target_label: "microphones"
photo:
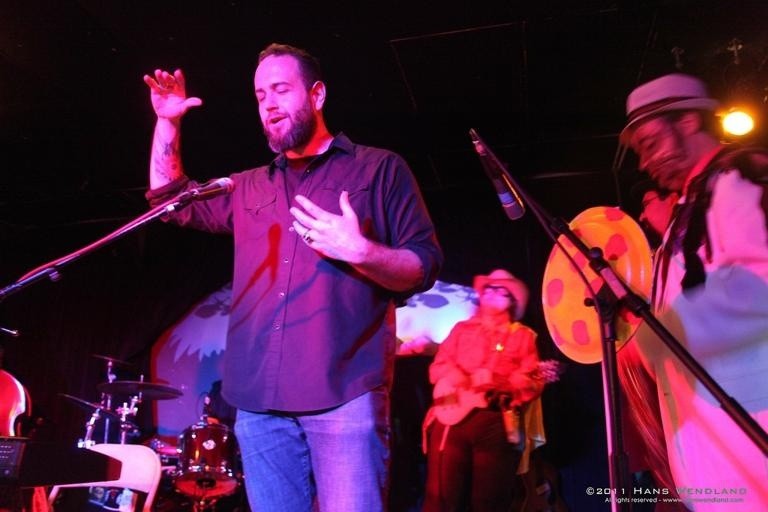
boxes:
[469,128,526,223]
[180,175,236,202]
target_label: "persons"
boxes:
[421,268,549,512]
[143,42,444,512]
[618,73,768,512]
[631,179,681,238]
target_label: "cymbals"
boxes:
[86,352,138,371]
[97,381,183,402]
[57,391,142,438]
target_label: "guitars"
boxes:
[432,360,560,427]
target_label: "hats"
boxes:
[473,269,530,320]
[620,74,722,144]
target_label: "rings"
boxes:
[302,233,316,246]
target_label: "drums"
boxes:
[149,439,188,500]
[174,422,244,498]
